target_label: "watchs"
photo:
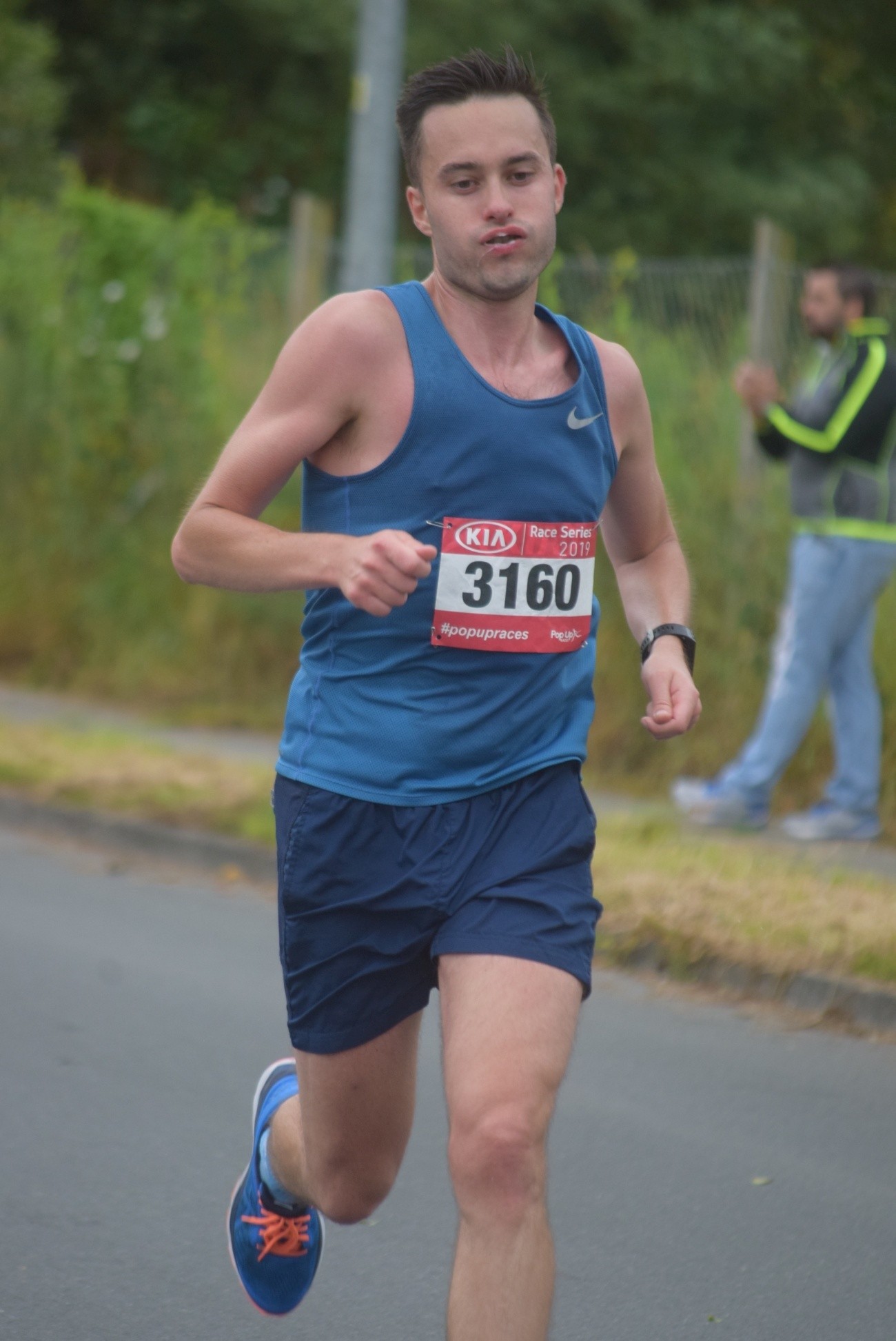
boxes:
[641,623,697,677]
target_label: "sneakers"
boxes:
[224,1058,325,1315]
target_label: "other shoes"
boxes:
[781,803,881,841]
[670,780,766,831]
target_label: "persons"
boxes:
[171,55,702,1341]
[675,264,896,841]
